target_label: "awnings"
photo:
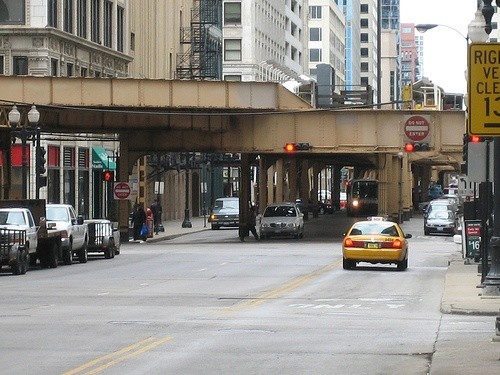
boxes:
[92,147,116,171]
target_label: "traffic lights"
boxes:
[101,171,114,182]
[405,143,431,152]
[35,146,46,174]
[469,136,483,143]
[285,143,310,151]
[38,175,47,187]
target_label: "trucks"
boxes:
[0,200,68,276]
[345,178,379,218]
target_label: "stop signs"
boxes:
[404,116,429,142]
[113,182,131,200]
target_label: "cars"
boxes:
[259,203,305,240]
[342,216,412,271]
[81,219,122,256]
[420,186,461,236]
[208,196,240,230]
[293,190,347,218]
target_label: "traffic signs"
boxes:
[457,177,480,197]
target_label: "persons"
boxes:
[240,208,260,241]
[150,199,162,235]
[130,204,147,241]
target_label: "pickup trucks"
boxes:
[46,205,91,266]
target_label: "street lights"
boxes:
[468,1,496,273]
[0,102,41,200]
